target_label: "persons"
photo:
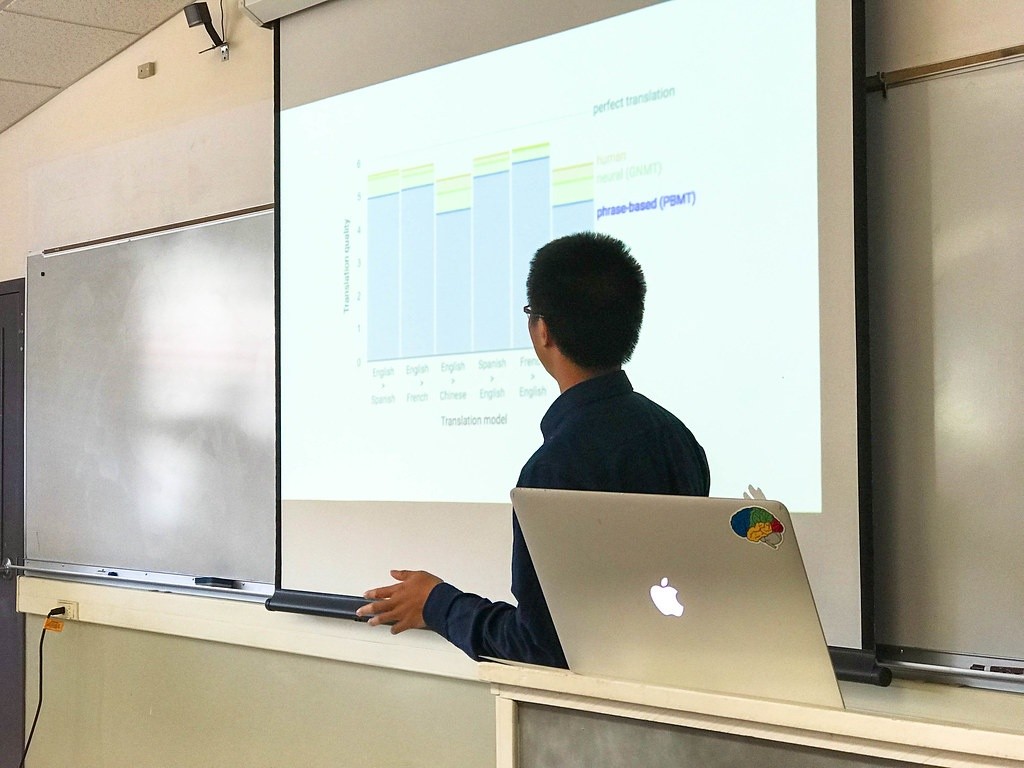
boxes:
[356,234,711,669]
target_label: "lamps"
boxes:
[182,1,229,55]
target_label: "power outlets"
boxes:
[137,61,154,79]
[56,599,79,622]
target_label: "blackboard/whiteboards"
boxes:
[22,202,280,596]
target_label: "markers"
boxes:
[190,575,246,589]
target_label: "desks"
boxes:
[485,662,1024,768]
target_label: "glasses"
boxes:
[524,303,547,322]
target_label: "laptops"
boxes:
[509,487,846,712]
